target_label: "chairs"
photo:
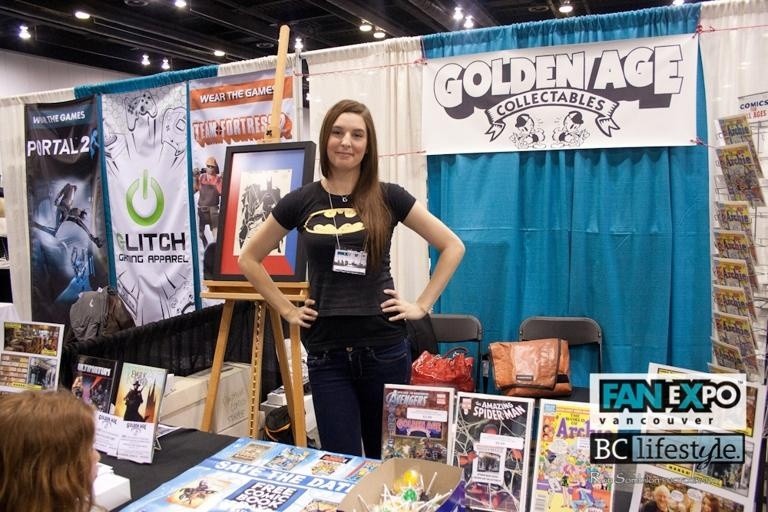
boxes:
[519,317,604,475]
[431,315,483,392]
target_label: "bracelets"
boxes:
[416,301,431,316]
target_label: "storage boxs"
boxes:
[186,360,252,433]
[158,376,208,429]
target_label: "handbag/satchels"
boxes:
[487,337,572,400]
[409,346,475,394]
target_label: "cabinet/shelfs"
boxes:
[711,120,768,385]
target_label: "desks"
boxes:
[98,429,239,512]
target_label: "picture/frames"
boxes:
[213,141,316,281]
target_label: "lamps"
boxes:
[174,0,186,8]
[142,53,150,67]
[161,56,170,71]
[19,24,31,40]
[295,36,303,49]
[360,6,474,40]
[558,0,573,13]
[213,50,227,57]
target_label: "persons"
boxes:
[238,99,466,459]
[0,389,99,511]
[191,157,223,247]
[123,380,146,422]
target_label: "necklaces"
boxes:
[324,176,358,203]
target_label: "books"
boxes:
[713,110,766,360]
[113,362,169,453]
[69,352,119,413]
[380,363,766,510]
